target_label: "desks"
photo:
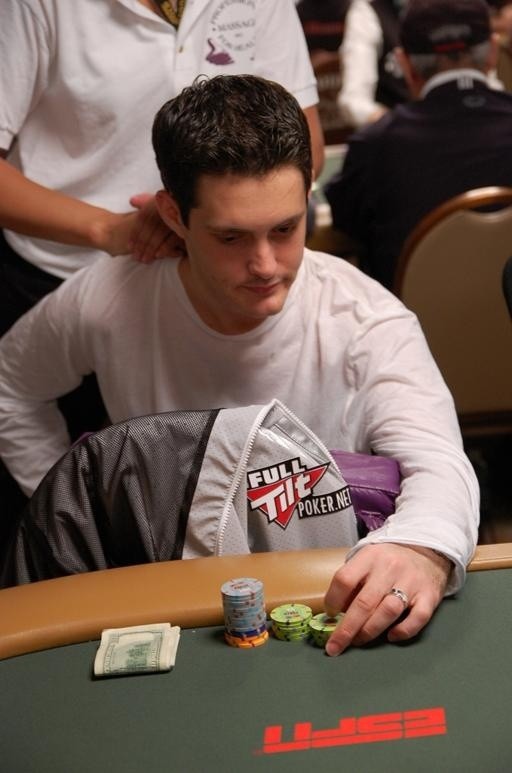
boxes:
[305,141,369,258]
[1,542,512,772]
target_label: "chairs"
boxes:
[391,184,511,439]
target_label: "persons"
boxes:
[313,0,512,326]
[0,68,484,661]
[0,0,327,583]
[332,0,500,131]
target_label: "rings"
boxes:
[391,586,412,610]
